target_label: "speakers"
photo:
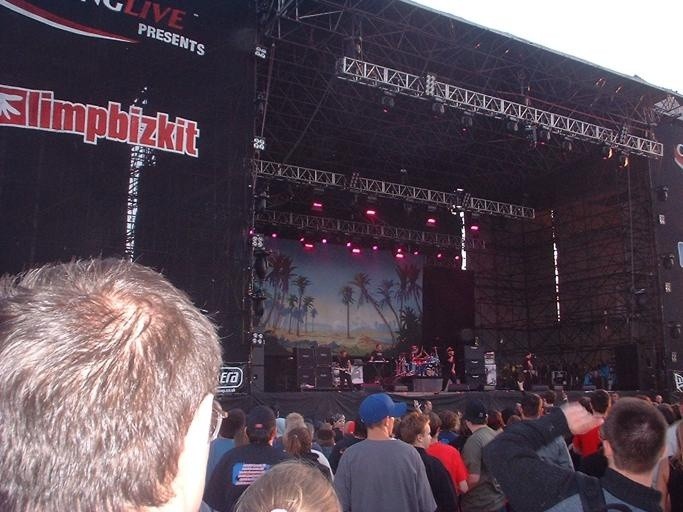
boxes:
[616,342,649,391]
[240,344,264,393]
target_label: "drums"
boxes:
[408,362,419,375]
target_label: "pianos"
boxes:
[368,360,389,365]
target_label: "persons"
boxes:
[421,411,468,495]
[652,402,683,488]
[431,344,440,377]
[634,394,682,425]
[409,343,417,375]
[440,345,457,392]
[497,350,579,391]
[334,392,437,512]
[207,400,228,442]
[1,256,223,512]
[487,406,506,435]
[482,397,668,512]
[332,417,366,476]
[305,413,331,431]
[274,411,308,451]
[439,412,467,451]
[413,399,423,414]
[656,418,682,512]
[368,343,386,391]
[328,414,346,443]
[203,404,294,512]
[328,420,355,475]
[572,389,612,478]
[388,418,402,438]
[553,383,568,405]
[205,408,246,489]
[579,361,619,390]
[305,431,323,454]
[517,380,526,396]
[457,407,466,419]
[424,399,433,413]
[518,392,574,469]
[507,415,520,426]
[400,412,461,512]
[283,426,334,484]
[514,399,523,414]
[501,406,521,426]
[610,391,621,403]
[235,460,342,512]
[317,421,335,460]
[461,398,507,511]
[331,350,356,394]
[270,404,280,418]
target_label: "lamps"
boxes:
[250,331,264,346]
[253,43,268,62]
[252,135,265,150]
[540,131,550,144]
[460,115,472,131]
[618,155,628,168]
[250,233,264,248]
[600,146,613,160]
[380,95,394,112]
[432,102,444,118]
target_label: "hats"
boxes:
[465,399,485,418]
[247,406,275,430]
[359,393,407,423]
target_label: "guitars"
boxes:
[333,367,352,375]
